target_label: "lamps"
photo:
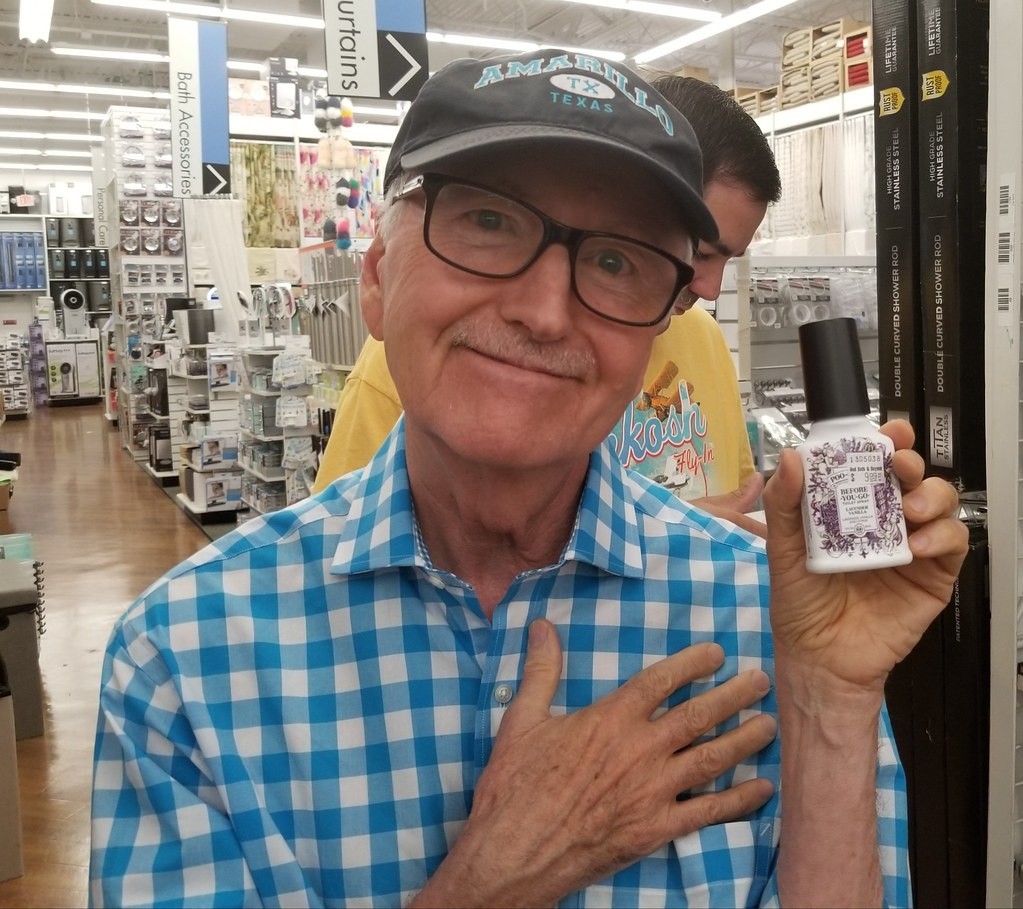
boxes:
[0,0,800,172]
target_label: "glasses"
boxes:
[390,175,695,328]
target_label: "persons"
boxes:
[212,483,225,500]
[88,51,970,909]
[208,442,221,458]
[215,364,229,379]
[311,76,780,543]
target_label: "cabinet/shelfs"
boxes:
[117,320,150,461]
[0,214,114,316]
[169,338,235,515]
[136,338,181,479]
[236,333,331,517]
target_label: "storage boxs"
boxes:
[669,18,873,121]
[0,332,35,410]
[0,482,12,511]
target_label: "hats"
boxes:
[381,48,721,245]
